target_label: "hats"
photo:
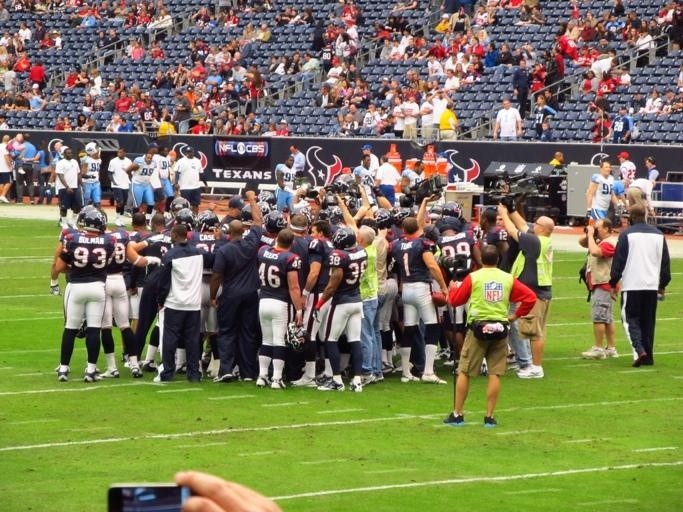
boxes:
[361,145,372,150]
[229,197,245,208]
[616,150,629,159]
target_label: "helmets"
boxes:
[331,225,356,249]
[290,201,343,225]
[242,191,288,233]
[284,322,310,353]
[171,196,219,231]
[84,143,100,155]
[78,205,108,233]
[373,207,412,229]
[431,201,463,219]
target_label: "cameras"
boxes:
[324,185,340,193]
[584,227,597,237]
[438,255,467,274]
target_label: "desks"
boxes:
[444,190,484,224]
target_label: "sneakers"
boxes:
[122,353,164,382]
[581,346,653,367]
[57,215,77,231]
[421,374,447,384]
[256,374,287,388]
[400,372,420,382]
[444,412,465,426]
[202,352,242,383]
[484,418,496,428]
[290,372,345,390]
[381,360,414,373]
[516,364,544,378]
[433,347,460,375]
[56,364,71,380]
[83,367,100,382]
[114,212,151,230]
[349,375,384,392]
[98,368,119,378]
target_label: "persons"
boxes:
[50,176,553,393]
[1,1,683,233]
[172,468,284,512]
[441,244,540,427]
[578,205,672,368]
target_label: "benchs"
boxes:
[200,179,247,205]
[257,181,278,199]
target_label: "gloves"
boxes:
[49,278,60,295]
[312,309,321,323]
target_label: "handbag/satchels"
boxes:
[472,321,509,340]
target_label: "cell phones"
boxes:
[108,483,191,512]
